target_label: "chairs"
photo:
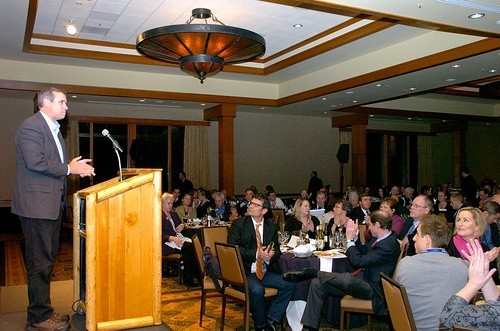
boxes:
[162,208,417,331]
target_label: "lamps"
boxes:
[135,8,266,85]
[66,19,77,35]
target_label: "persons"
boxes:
[325,198,358,242]
[173,172,193,203]
[307,171,324,195]
[161,180,500,331]
[11,87,95,331]
[363,198,405,240]
[284,198,320,240]
[460,166,478,202]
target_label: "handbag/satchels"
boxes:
[282,267,317,281]
[202,247,222,280]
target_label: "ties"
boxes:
[404,224,415,238]
[351,240,378,276]
[255,224,264,280]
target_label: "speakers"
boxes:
[336,143,350,163]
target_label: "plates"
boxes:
[312,251,335,258]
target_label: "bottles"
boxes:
[314,223,329,250]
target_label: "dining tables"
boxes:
[180,220,233,279]
[268,242,348,331]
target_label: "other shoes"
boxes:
[185,276,195,287]
[255,320,276,331]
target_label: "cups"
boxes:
[278,230,347,253]
[182,216,225,227]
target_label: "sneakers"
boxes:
[51,312,70,322]
[26,318,71,331]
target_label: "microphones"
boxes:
[101,128,124,153]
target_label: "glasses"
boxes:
[410,202,427,209]
[248,201,266,209]
